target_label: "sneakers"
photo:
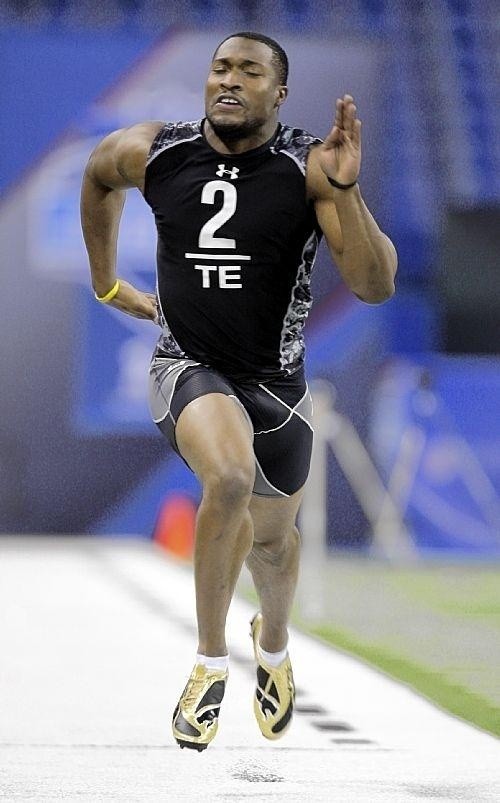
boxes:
[249,609,296,743]
[170,662,230,753]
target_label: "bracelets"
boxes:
[89,278,121,303]
[328,176,358,189]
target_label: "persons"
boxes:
[76,31,402,754]
